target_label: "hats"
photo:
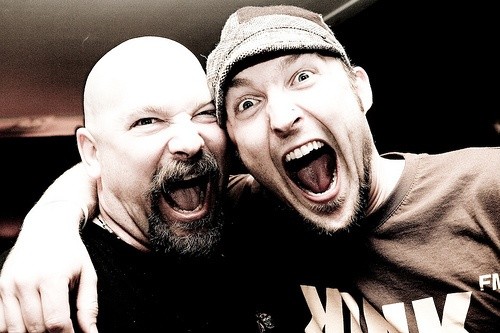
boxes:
[204,5,353,129]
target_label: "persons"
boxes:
[1,5,499,333]
[69,36,295,333]
